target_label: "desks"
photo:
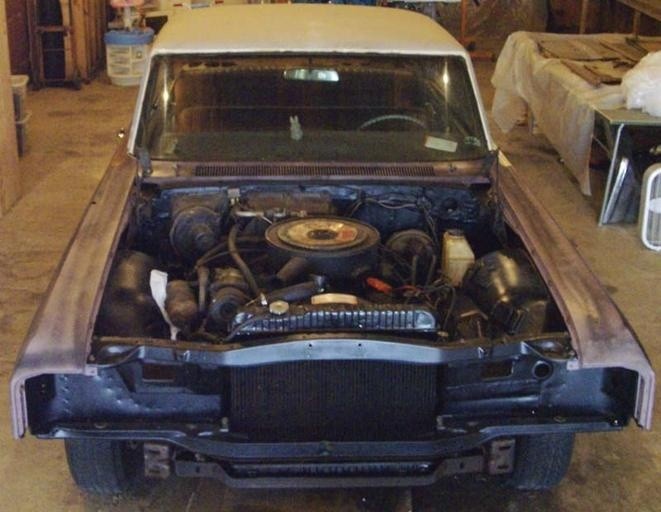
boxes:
[517,32,660,226]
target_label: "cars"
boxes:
[11,2,657,496]
